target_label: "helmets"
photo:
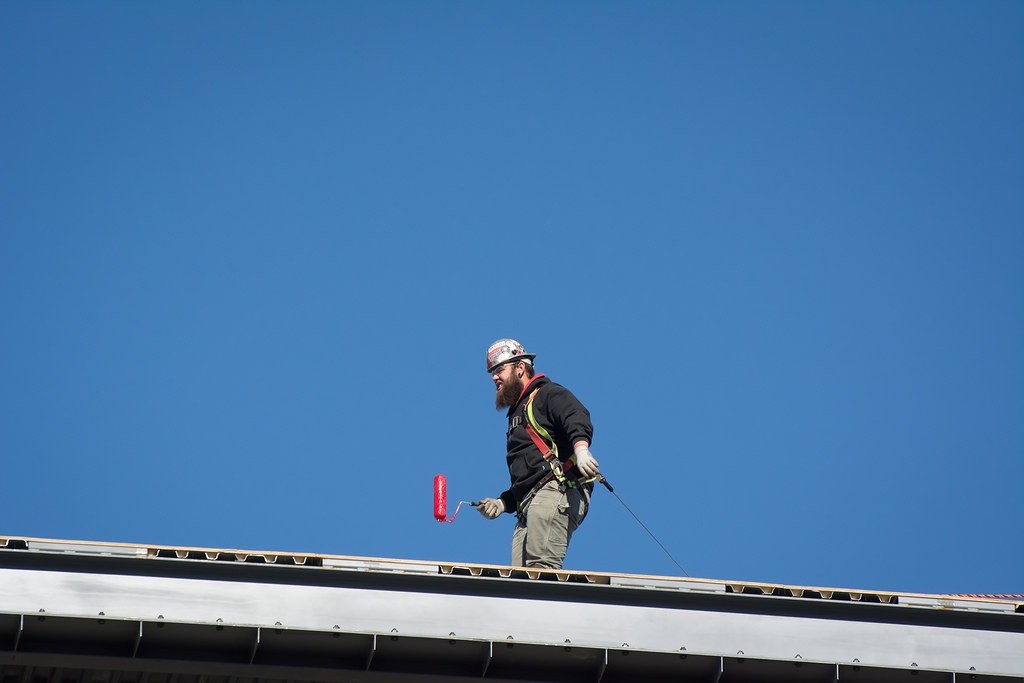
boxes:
[486,339,535,372]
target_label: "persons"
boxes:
[477,338,599,570]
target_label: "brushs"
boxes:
[432,473,481,523]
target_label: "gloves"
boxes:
[574,445,599,479]
[476,498,507,520]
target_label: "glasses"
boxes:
[492,362,516,375]
